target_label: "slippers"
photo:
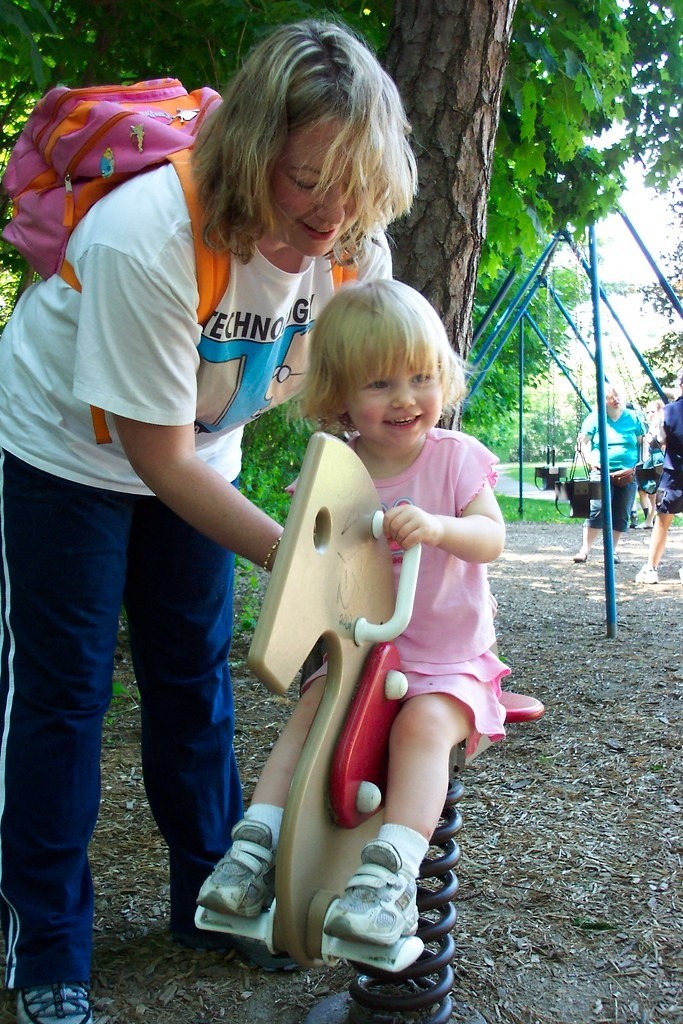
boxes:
[635,523,655,528]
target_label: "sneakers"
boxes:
[636,564,659,584]
[196,819,278,918]
[16,981,94,1024]
[324,839,419,947]
[174,932,300,970]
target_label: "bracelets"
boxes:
[261,532,290,575]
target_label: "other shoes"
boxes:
[613,553,620,563]
[573,552,588,562]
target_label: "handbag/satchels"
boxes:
[593,466,638,488]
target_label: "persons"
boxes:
[0,14,421,1024]
[198,277,507,946]
[634,377,683,587]
[571,383,647,569]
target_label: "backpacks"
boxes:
[0,77,359,445]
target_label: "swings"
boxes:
[534,261,566,492]
[554,225,602,521]
[574,265,665,494]
